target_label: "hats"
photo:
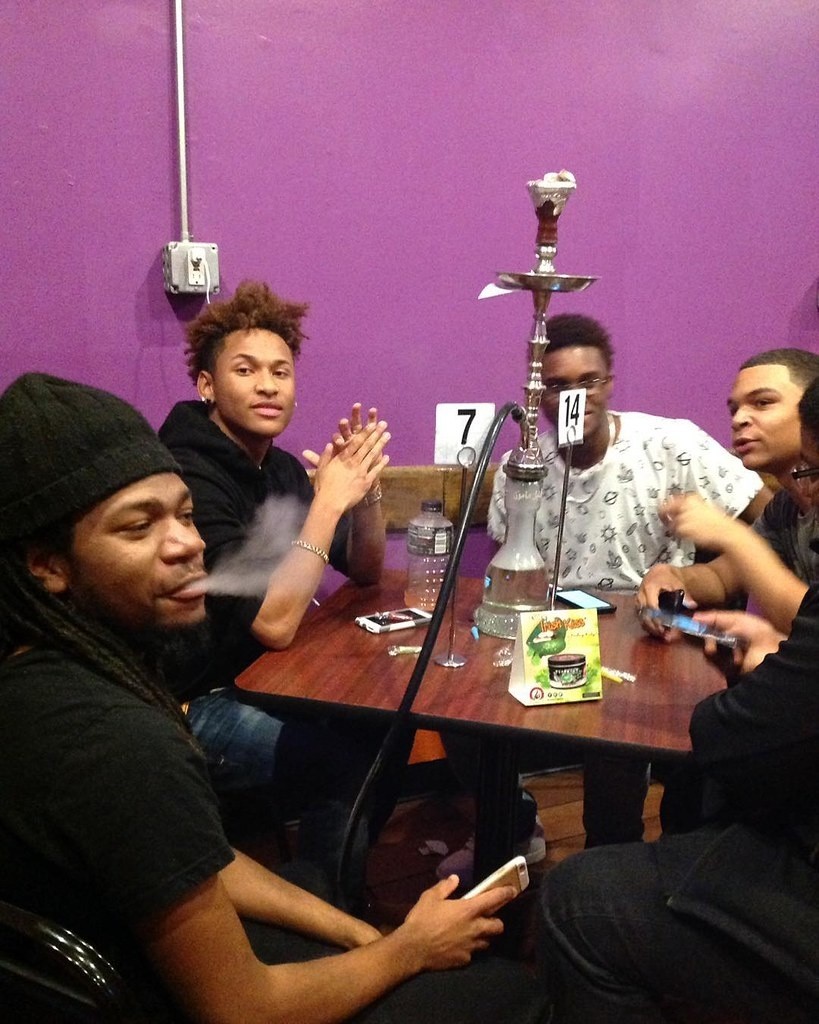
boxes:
[1,371,183,567]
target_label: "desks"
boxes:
[233,573,729,899]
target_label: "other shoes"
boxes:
[331,873,377,919]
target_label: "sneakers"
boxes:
[436,814,547,881]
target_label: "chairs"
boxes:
[0,902,143,1024]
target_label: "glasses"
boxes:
[540,376,613,403]
[790,461,819,489]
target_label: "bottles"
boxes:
[404,500,453,613]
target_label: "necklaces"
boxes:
[567,412,616,503]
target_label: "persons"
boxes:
[435,309,771,887]
[158,278,419,913]
[0,370,520,1024]
[535,374,819,1024]
[634,348,819,664]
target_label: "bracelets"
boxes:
[353,485,382,509]
[291,540,329,564]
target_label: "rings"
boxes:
[664,513,672,522]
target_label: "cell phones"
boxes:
[353,607,434,634]
[457,855,530,916]
[658,589,685,630]
[662,612,737,649]
[550,588,617,615]
